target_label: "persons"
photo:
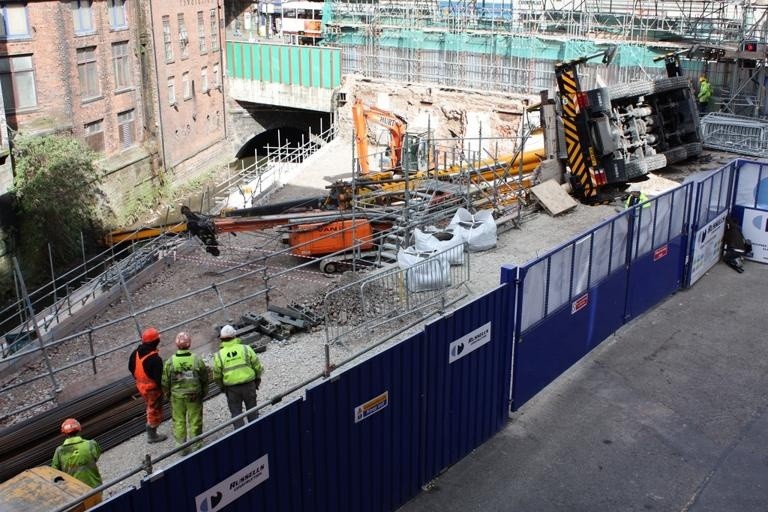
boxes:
[159,332,208,456]
[51,418,103,497]
[212,324,263,430]
[128,327,168,444]
[698,76,714,117]
[751,66,768,118]
[624,183,651,211]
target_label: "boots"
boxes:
[146,424,204,456]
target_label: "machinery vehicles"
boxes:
[290,98,435,273]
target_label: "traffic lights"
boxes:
[741,41,757,69]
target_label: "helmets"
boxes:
[628,183,642,192]
[61,418,81,433]
[175,331,192,348]
[221,325,236,338]
[142,328,160,344]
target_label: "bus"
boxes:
[282,3,431,45]
